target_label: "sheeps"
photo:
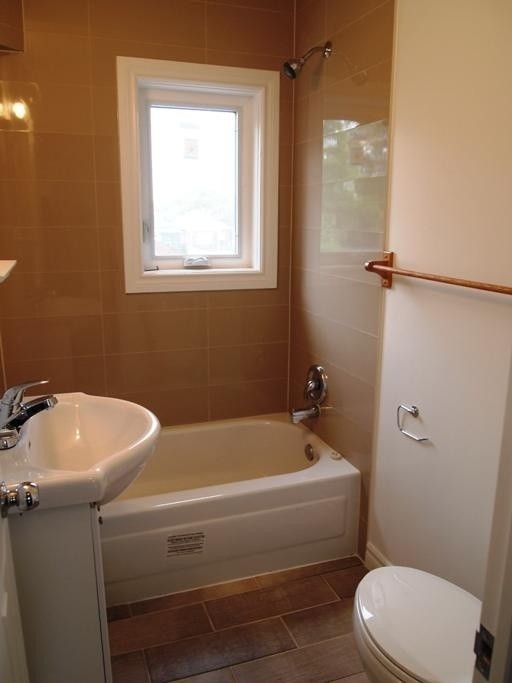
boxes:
[289,403,320,425]
[1,380,58,447]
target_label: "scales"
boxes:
[100,411,361,622]
